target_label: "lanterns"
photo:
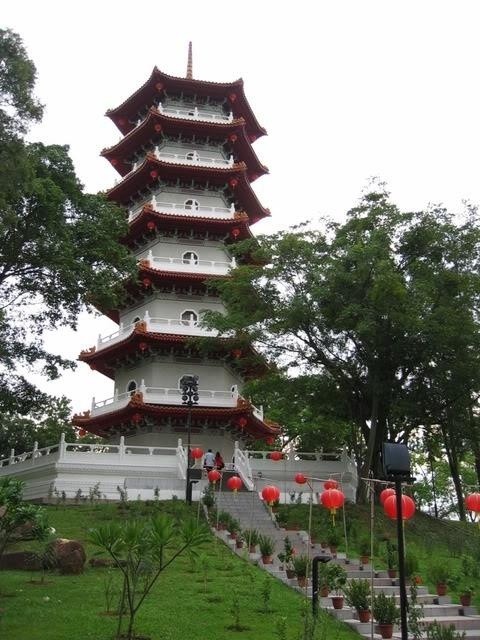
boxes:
[295,472,306,484]
[106,112,128,170]
[324,480,338,490]
[208,470,221,484]
[262,485,280,506]
[227,474,242,494]
[265,432,277,447]
[384,495,416,520]
[321,489,344,527]
[271,451,281,460]
[131,80,168,426]
[250,128,265,228]
[75,426,90,438]
[226,83,249,445]
[466,491,479,512]
[380,488,396,504]
[192,448,202,459]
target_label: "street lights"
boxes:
[379,442,411,640]
[179,374,199,504]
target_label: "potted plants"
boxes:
[316,560,336,597]
[258,535,278,564]
[271,498,301,533]
[275,537,296,578]
[329,564,348,610]
[459,585,474,607]
[409,561,421,587]
[310,520,341,554]
[359,542,372,565]
[205,486,239,539]
[372,590,467,637]
[235,529,246,549]
[243,527,260,553]
[291,554,312,588]
[387,546,399,578]
[344,578,373,623]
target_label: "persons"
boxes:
[214,452,224,470]
[204,449,215,471]
